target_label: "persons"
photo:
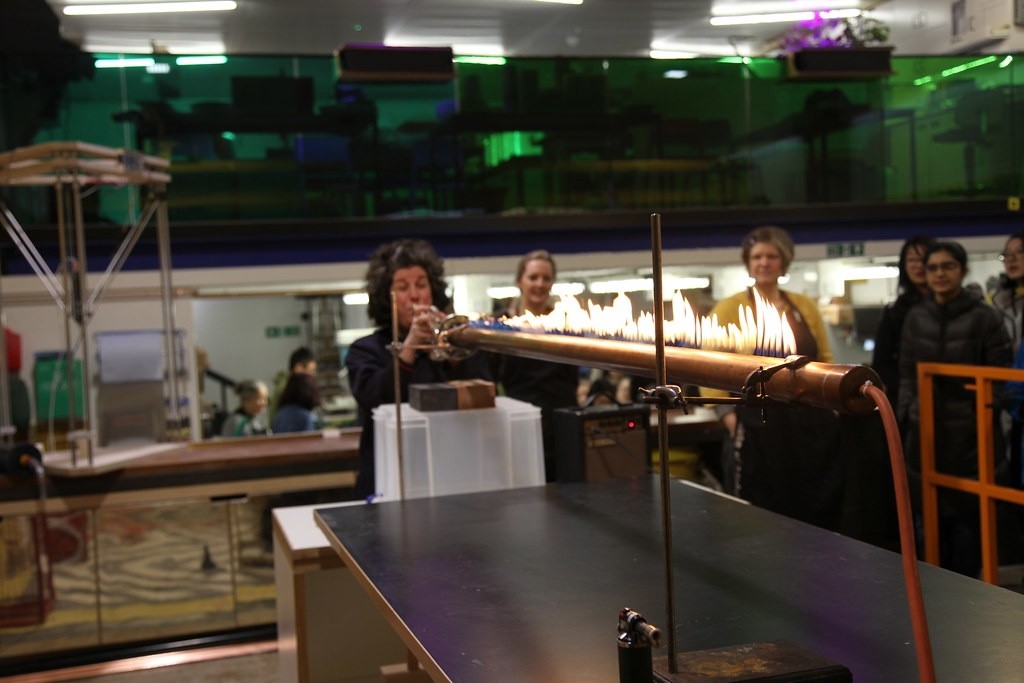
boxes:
[474,248,580,488]
[271,372,323,434]
[896,240,1014,575]
[982,230,1024,566]
[220,377,274,437]
[584,367,630,410]
[345,239,499,501]
[289,346,317,376]
[697,226,836,498]
[867,232,940,546]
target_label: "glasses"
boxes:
[927,263,959,271]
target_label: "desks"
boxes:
[272,500,413,683]
[316,477,1024,683]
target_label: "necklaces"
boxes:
[516,298,551,319]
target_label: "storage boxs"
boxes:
[373,396,545,502]
